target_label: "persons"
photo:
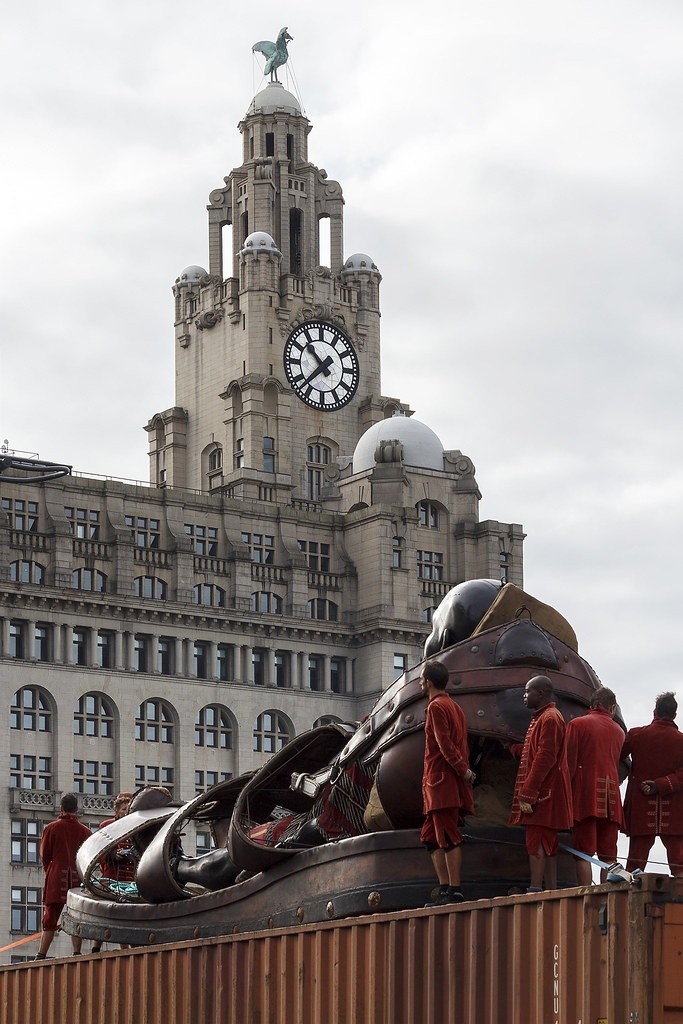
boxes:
[93,792,140,953]
[33,793,93,962]
[127,579,630,892]
[419,661,478,908]
[509,676,575,895]
[622,690,683,876]
[568,687,625,887]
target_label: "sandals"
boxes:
[57,605,631,947]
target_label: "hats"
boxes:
[115,792,133,803]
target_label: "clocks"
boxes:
[284,319,360,412]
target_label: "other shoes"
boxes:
[442,890,463,904]
[92,947,100,953]
[72,952,82,956]
[34,952,46,961]
[425,892,446,906]
